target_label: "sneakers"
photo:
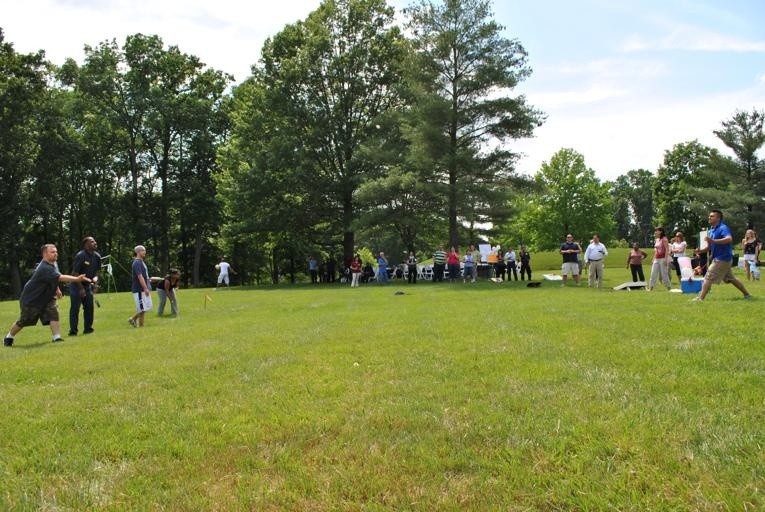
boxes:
[127,317,137,327]
[689,296,704,301]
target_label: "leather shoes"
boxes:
[4,337,14,347]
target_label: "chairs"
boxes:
[363,260,466,281]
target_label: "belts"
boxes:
[588,258,602,262]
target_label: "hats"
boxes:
[134,244,146,254]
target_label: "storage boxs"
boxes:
[681,278,705,293]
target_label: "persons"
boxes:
[626,242,648,282]
[649,209,762,301]
[305,244,531,287]
[156,268,181,316]
[215,260,238,287]
[128,245,153,327]
[68,236,101,336]
[559,233,609,289]
[4,244,92,346]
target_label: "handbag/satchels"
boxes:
[752,270,760,280]
